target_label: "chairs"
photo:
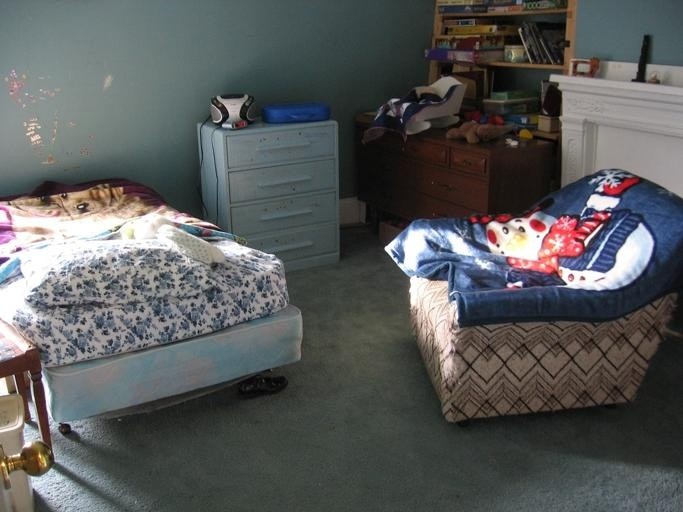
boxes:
[407,166,682,425]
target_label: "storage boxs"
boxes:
[536,114,559,132]
[503,44,530,63]
[379,212,413,249]
[481,97,539,116]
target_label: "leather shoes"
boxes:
[237,375,288,399]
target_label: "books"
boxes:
[424,0,567,65]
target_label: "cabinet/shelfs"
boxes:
[195,119,340,276]
[424,1,578,118]
[353,110,556,226]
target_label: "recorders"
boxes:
[210,94,257,128]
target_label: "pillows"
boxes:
[18,239,202,309]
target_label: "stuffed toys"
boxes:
[446,122,517,144]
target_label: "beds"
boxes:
[2,179,305,435]
